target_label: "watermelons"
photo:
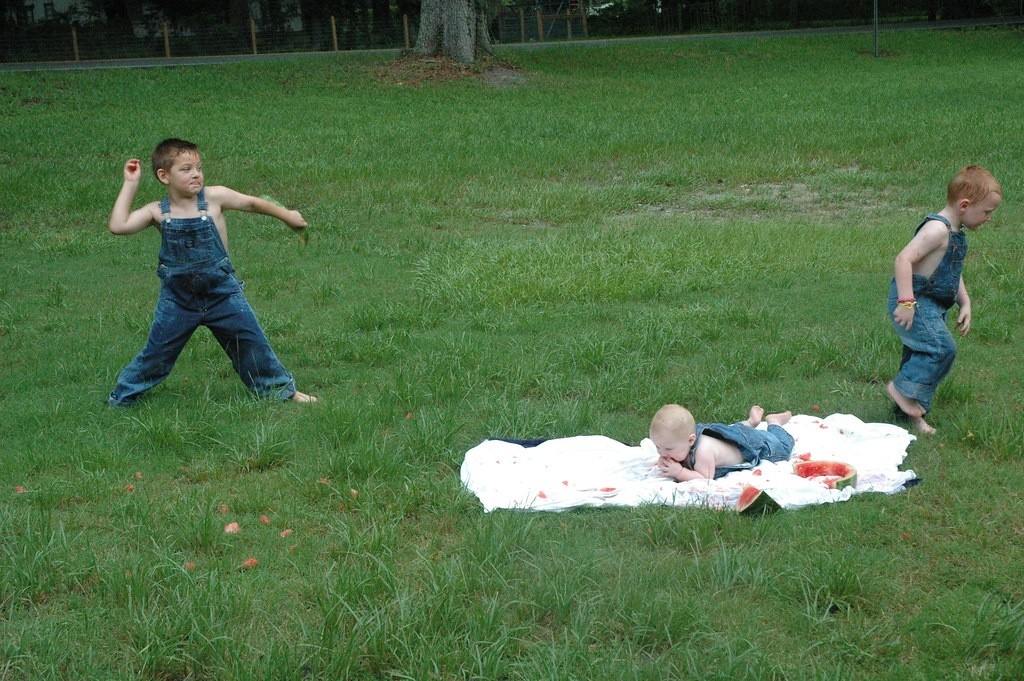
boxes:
[15,472,359,571]
[738,484,782,514]
[538,481,616,498]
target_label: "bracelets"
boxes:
[897,297,918,309]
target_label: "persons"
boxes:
[886,164,1002,436]
[108,138,317,405]
[650,403,794,482]
